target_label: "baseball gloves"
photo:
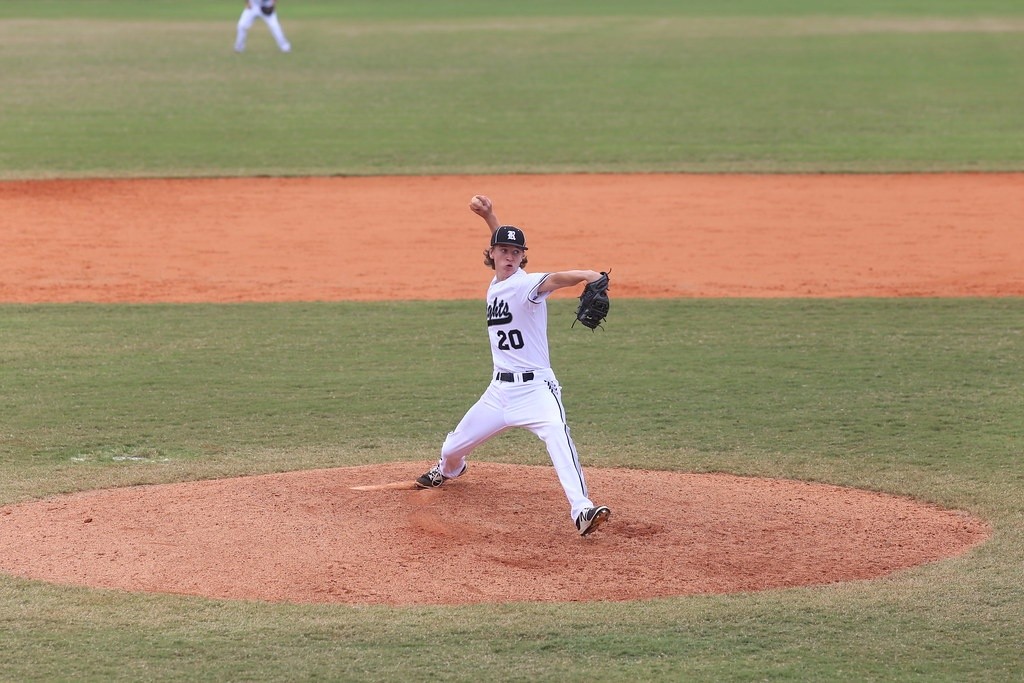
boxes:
[571,268,611,332]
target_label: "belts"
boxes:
[496,372,534,382]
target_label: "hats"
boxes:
[490,225,528,250]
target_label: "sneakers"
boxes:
[415,462,467,488]
[575,505,610,536]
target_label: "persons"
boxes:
[416,195,610,538]
[234,0,291,54]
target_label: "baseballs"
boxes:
[471,196,484,210]
